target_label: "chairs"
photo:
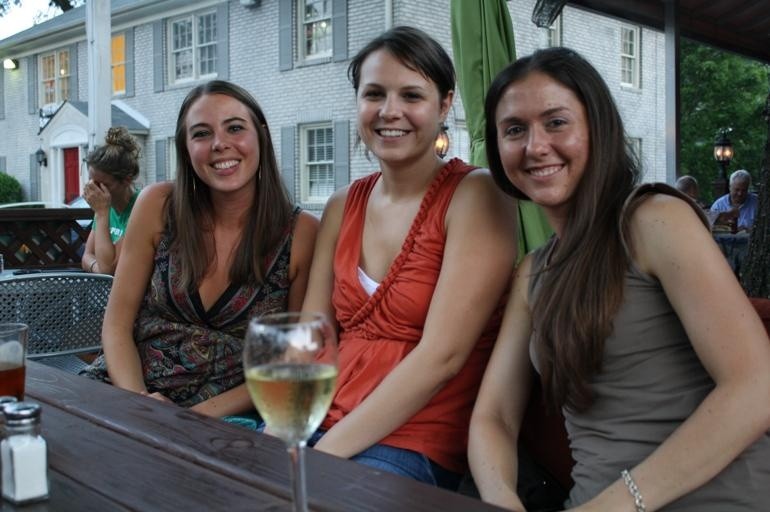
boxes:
[748,297,770,337]
[0,274,113,378]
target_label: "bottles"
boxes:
[0,395,51,507]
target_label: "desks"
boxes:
[710,228,752,271]
[0,358,534,512]
[0,268,93,330]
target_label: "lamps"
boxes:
[3,58,18,71]
[35,146,47,167]
[83,142,97,170]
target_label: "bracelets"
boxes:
[91,260,96,273]
[619,469,646,512]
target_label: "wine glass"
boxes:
[243,311,338,512]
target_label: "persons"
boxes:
[74,81,323,434]
[74,125,145,363]
[255,24,521,487]
[708,169,759,272]
[465,44,769,511]
[675,175,740,225]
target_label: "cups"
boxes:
[0,322,29,402]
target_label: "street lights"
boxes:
[713,129,735,200]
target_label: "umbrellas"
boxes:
[449,0,566,268]
